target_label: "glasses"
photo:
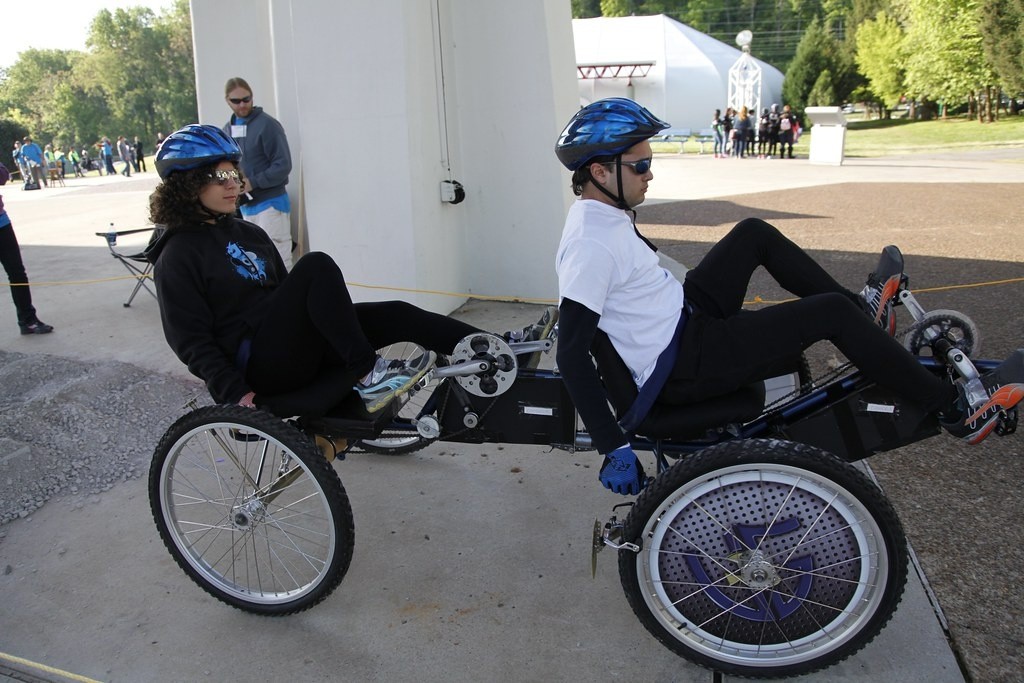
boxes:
[586,157,652,174]
[209,167,240,185]
[229,96,251,104]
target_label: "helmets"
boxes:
[556,96,672,171]
[154,123,243,179]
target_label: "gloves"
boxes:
[599,442,647,494]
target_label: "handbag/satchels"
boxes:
[24,180,40,190]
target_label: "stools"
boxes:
[46,167,66,188]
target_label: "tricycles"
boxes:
[146,273,1023,679]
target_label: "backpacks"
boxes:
[780,117,791,130]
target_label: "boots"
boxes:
[780,149,784,158]
[788,147,795,158]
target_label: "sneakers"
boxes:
[505,306,559,368]
[859,244,904,338]
[939,350,1024,444]
[21,321,53,335]
[353,350,437,413]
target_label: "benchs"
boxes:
[647,137,688,153]
[695,138,715,154]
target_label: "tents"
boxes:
[572,14,785,137]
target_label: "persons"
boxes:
[223,76,292,272]
[13,132,169,190]
[0,162,53,333]
[711,103,798,160]
[555,96,1023,497]
[150,124,558,415]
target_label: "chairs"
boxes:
[95,222,166,307]
